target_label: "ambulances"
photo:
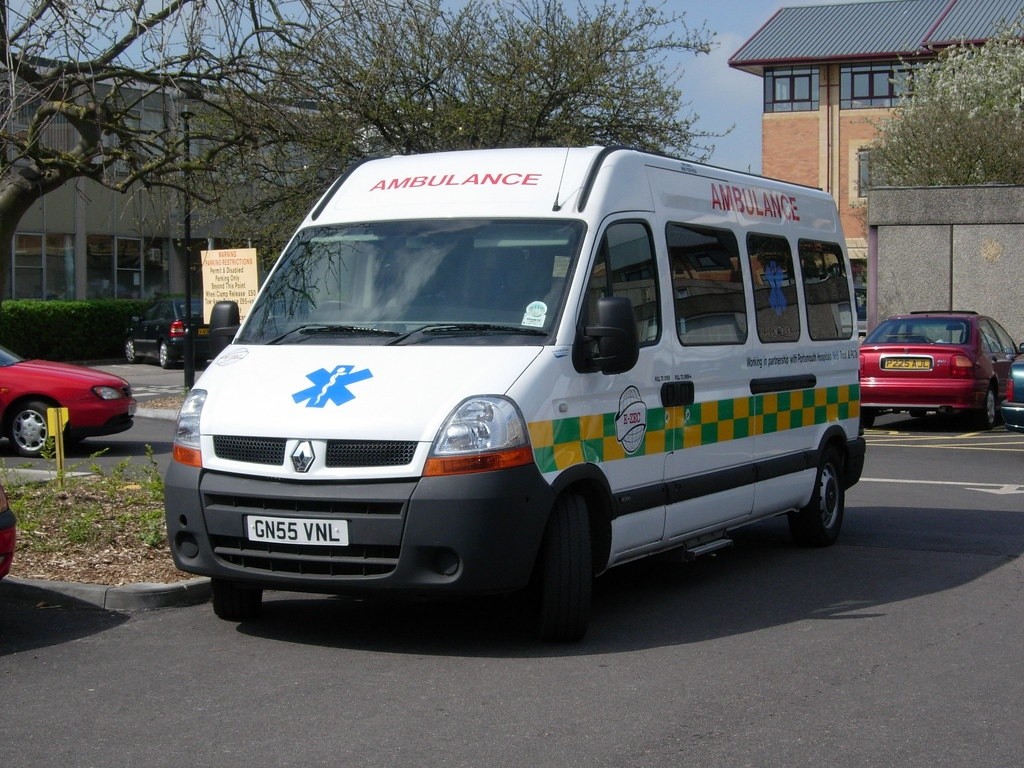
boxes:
[163,145,866,648]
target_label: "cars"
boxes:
[859,310,1019,430]
[1000,342,1024,434]
[0,345,137,458]
[123,297,210,369]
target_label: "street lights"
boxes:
[167,70,205,394]
